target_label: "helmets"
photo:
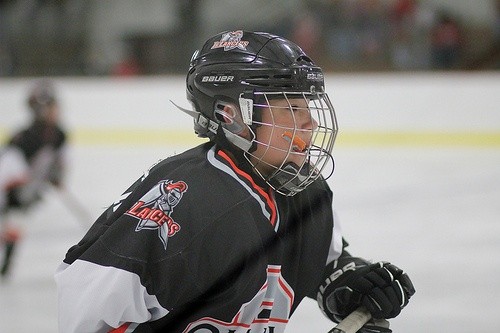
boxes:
[185,31,325,149]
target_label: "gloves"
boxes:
[314,256,414,333]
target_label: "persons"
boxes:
[0,75,78,284]
[0,0,500,69]
[52,28,418,333]
